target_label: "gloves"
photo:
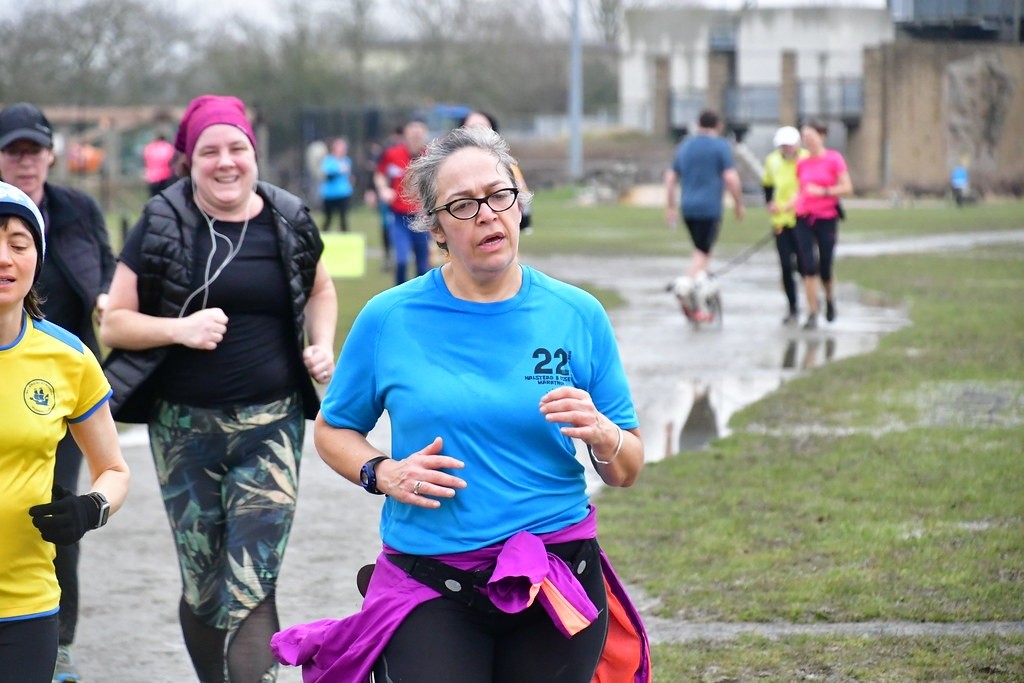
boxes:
[29,484,101,544]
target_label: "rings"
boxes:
[413,481,421,494]
[323,371,328,379]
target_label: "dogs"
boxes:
[669,273,720,325]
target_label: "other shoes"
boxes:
[805,309,820,326]
[825,296,838,321]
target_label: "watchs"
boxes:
[360,456,389,497]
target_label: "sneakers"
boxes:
[52,645,79,683]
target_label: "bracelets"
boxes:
[590,424,623,465]
[823,186,830,195]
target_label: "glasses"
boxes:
[426,187,519,220]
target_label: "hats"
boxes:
[0,103,52,150]
[773,126,800,147]
[1,181,45,262]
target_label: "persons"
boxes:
[269,123,653,683]
[792,120,853,331]
[459,111,531,231]
[101,94,338,683]
[375,118,432,284]
[764,126,810,324]
[951,158,968,205]
[666,111,745,322]
[144,132,175,196]
[0,102,117,683]
[366,126,407,256]
[0,181,131,683]
[319,136,356,232]
